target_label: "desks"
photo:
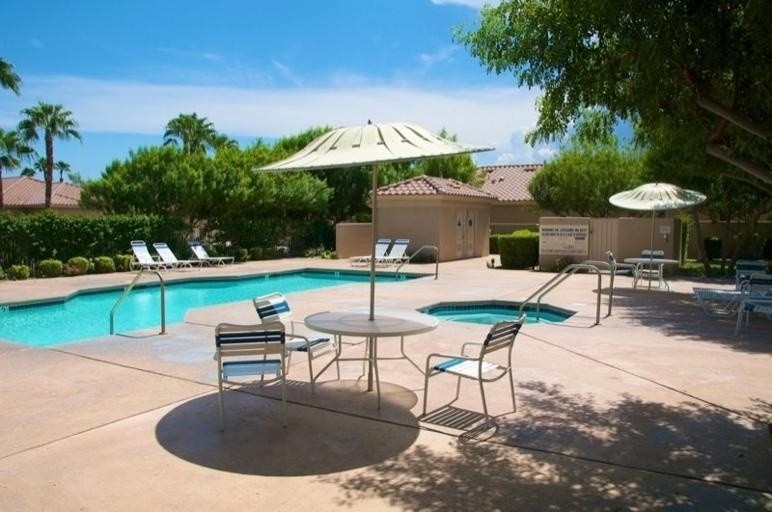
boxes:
[304,308,439,410]
[624,258,679,292]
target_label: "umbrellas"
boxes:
[609,182,707,292]
[251,120,496,390]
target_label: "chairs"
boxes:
[637,250,665,287]
[153,243,205,271]
[375,238,411,267]
[253,292,340,394]
[423,314,527,428]
[213,321,290,430]
[130,239,178,272]
[349,238,392,268]
[693,260,772,335]
[187,241,234,267]
